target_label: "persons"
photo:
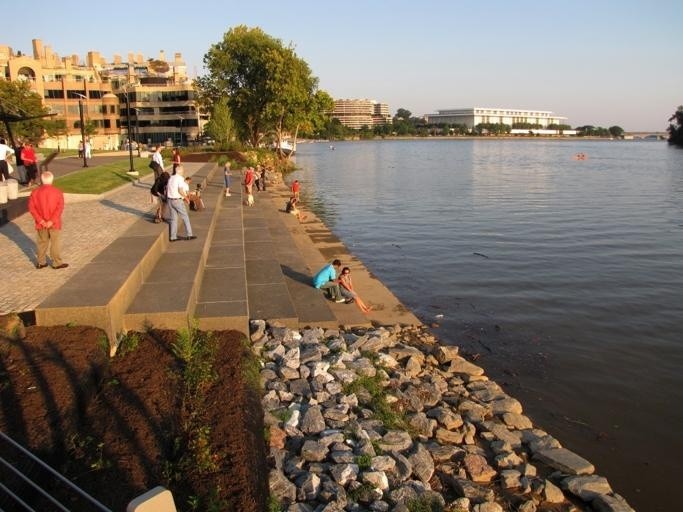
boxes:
[28,171,69,268]
[337,266,373,313]
[125,136,129,151]
[244,166,254,206]
[286,197,303,219]
[77,137,93,158]
[291,180,300,200]
[148,143,205,241]
[254,167,260,191]
[223,161,232,197]
[246,191,254,207]
[260,168,267,191]
[0,138,38,187]
[312,259,345,303]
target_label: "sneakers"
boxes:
[334,298,345,303]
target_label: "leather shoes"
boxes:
[36,262,50,270]
[187,236,197,240]
[170,237,181,242]
[51,263,68,270]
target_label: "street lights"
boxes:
[121,80,141,172]
[176,114,184,146]
[73,93,89,168]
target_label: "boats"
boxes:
[571,154,589,161]
[271,141,295,155]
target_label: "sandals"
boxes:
[364,305,372,315]
